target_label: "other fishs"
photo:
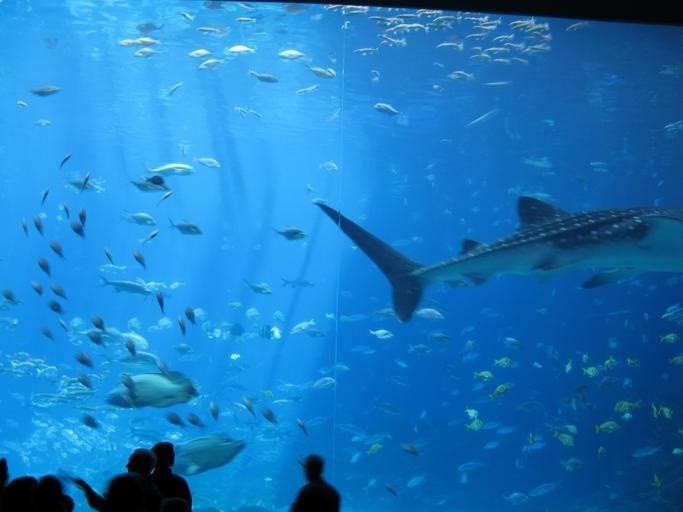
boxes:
[0,1,683,512]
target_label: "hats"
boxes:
[126,441,174,469]
[296,453,325,476]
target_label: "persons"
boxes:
[289,453,341,512]
[1,441,192,512]
